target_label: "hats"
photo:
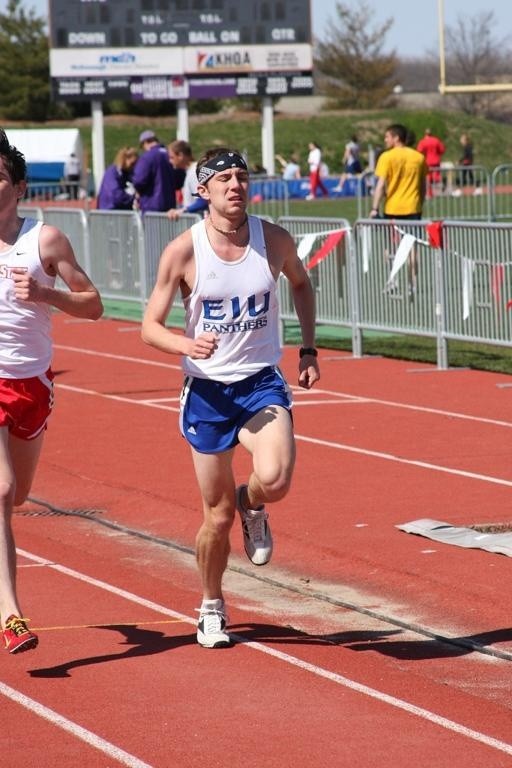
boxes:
[139,129,155,145]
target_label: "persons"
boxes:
[0,132,105,654]
[141,143,322,650]
[63,152,82,200]
[94,124,215,304]
[236,124,477,200]
[368,126,426,300]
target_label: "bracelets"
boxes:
[297,345,321,362]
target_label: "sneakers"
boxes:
[472,187,481,195]
[381,277,413,301]
[2,616,38,654]
[234,484,273,566]
[332,186,342,192]
[196,599,230,649]
[305,194,316,200]
[452,188,462,196]
